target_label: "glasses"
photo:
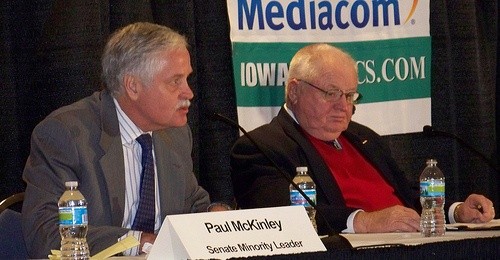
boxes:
[295,79,362,105]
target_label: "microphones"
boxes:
[423,126,500,174]
[212,112,354,251]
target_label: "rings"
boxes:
[492,207,494,208]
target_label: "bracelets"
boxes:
[207,203,231,212]
[455,204,464,223]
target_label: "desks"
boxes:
[107,219,500,260]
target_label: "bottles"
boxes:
[420,159,446,237]
[58,182,89,260]
[290,167,317,233]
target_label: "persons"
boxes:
[21,22,233,259]
[228,43,496,236]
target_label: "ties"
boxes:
[131,133,155,235]
